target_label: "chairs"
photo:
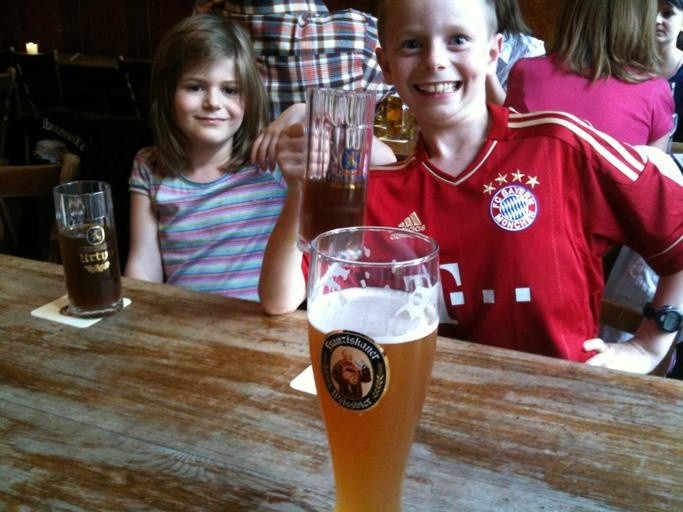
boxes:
[0,47,153,263]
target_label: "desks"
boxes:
[0,253,683,510]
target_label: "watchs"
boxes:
[641,301,682,334]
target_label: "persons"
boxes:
[650,0,682,143]
[487,1,548,111]
[189,1,393,125]
[120,12,402,305]
[255,2,682,376]
[499,2,676,154]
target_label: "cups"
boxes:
[305,225,442,512]
[53,178,125,320]
[386,95,415,140]
[295,85,378,265]
